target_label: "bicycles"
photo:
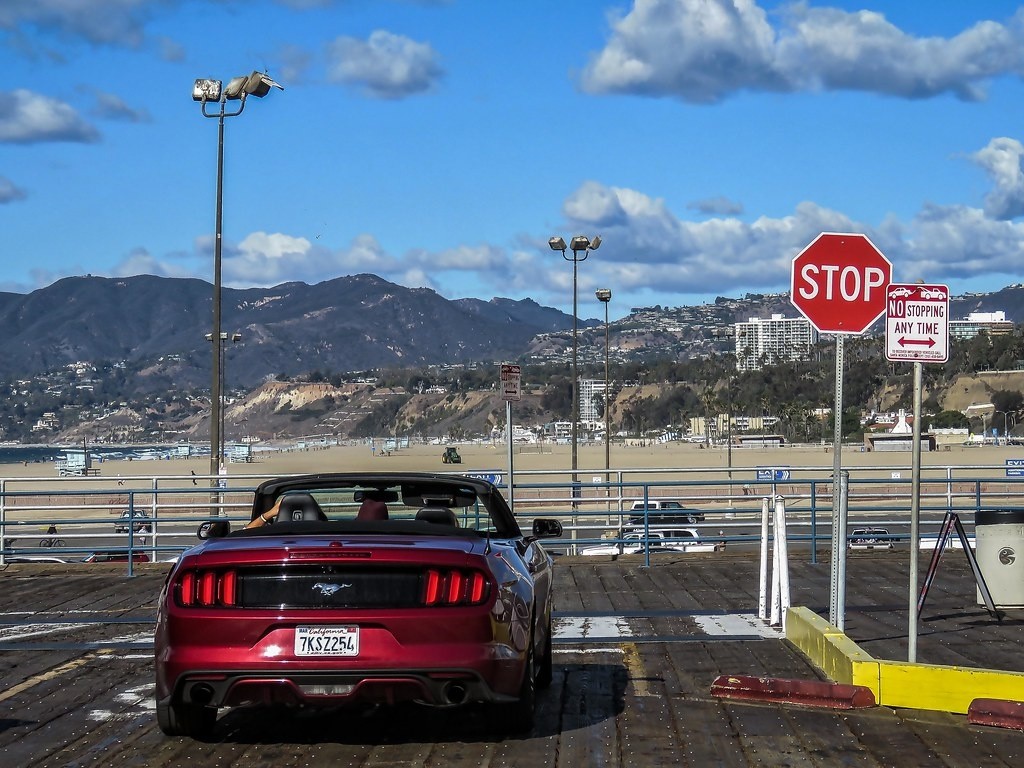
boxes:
[139,536,146,547]
[40,533,66,547]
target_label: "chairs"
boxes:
[275,492,328,524]
[415,506,460,528]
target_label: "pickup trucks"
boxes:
[114,510,152,533]
[847,528,895,550]
[578,532,716,552]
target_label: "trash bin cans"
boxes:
[974,503,1024,616]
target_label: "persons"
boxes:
[742,484,752,495]
[117,474,124,486]
[191,470,197,485]
[240,493,287,530]
[714,530,728,552]
[138,526,148,545]
[46,524,57,545]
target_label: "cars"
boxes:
[4,555,87,563]
[79,550,151,562]
[622,509,698,525]
[631,548,684,553]
[633,529,704,545]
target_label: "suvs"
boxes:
[630,499,705,521]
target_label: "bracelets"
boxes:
[260,514,268,523]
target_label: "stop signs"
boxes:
[790,231,892,335]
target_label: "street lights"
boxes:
[193,68,283,518]
[549,235,602,556]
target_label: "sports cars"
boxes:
[154,471,554,734]
